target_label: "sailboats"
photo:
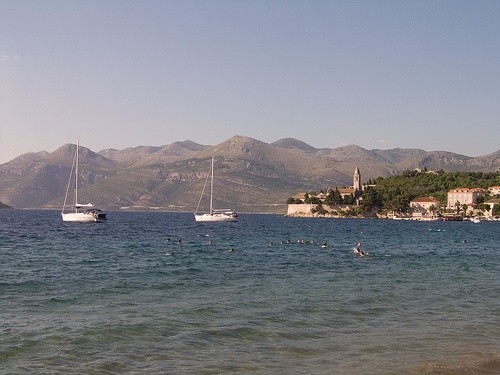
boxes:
[195,157,238,223]
[60,138,105,221]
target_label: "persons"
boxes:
[164,227,469,258]
